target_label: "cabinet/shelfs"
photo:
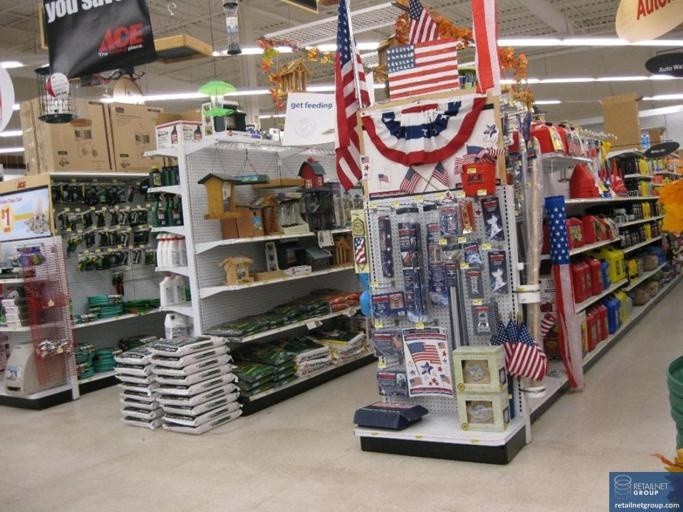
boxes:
[0,135,683,466]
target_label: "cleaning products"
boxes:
[155,233,186,308]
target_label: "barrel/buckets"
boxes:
[529,119,630,358]
[165,314,188,341]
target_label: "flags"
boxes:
[405,0,441,43]
[378,173,389,184]
[433,161,450,187]
[489,317,549,382]
[409,376,423,388]
[406,341,440,366]
[383,38,460,101]
[453,145,498,176]
[333,1,370,193]
[398,167,420,196]
[440,374,450,385]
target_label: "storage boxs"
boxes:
[21,95,164,175]
